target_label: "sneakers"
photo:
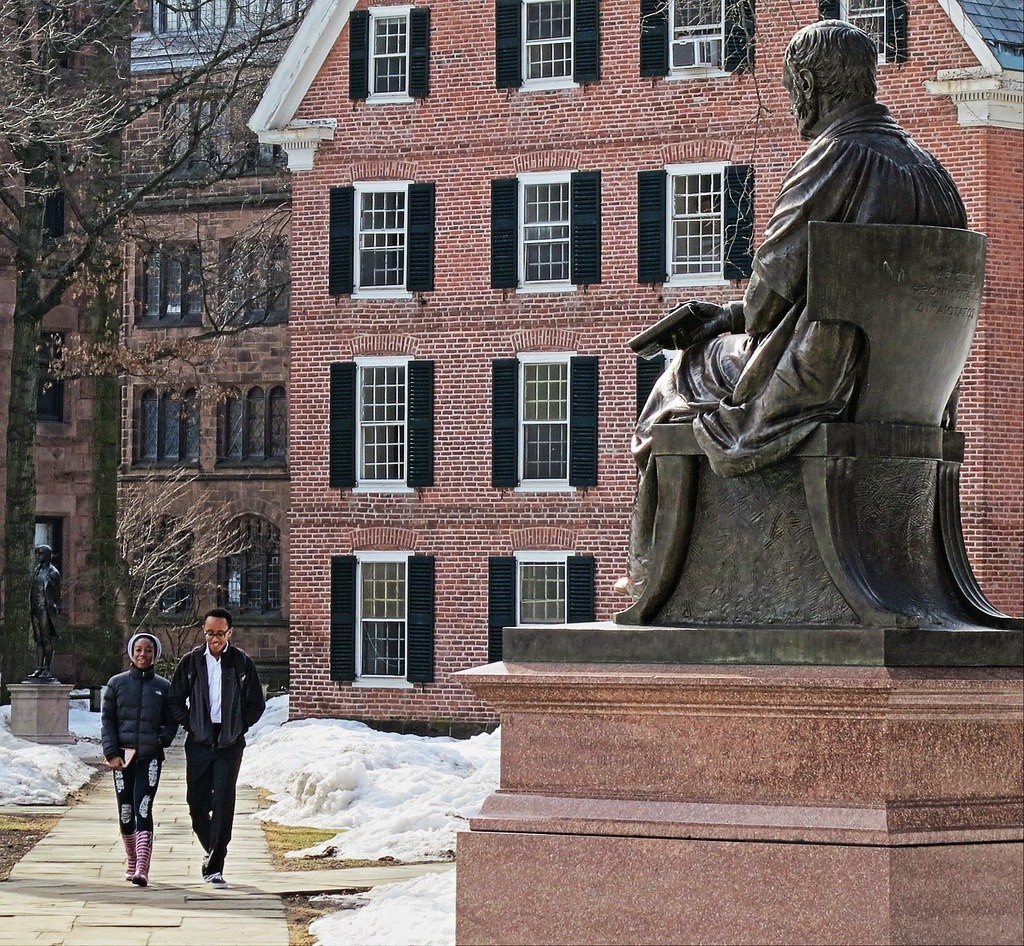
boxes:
[206,872,226,890]
[201,850,212,877]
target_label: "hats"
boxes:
[128,633,162,669]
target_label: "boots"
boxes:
[132,830,153,888]
[120,829,138,881]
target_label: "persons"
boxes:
[632,20,968,631]
[27,543,61,678]
[168,607,265,889]
[101,633,179,887]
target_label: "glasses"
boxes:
[203,626,229,639]
